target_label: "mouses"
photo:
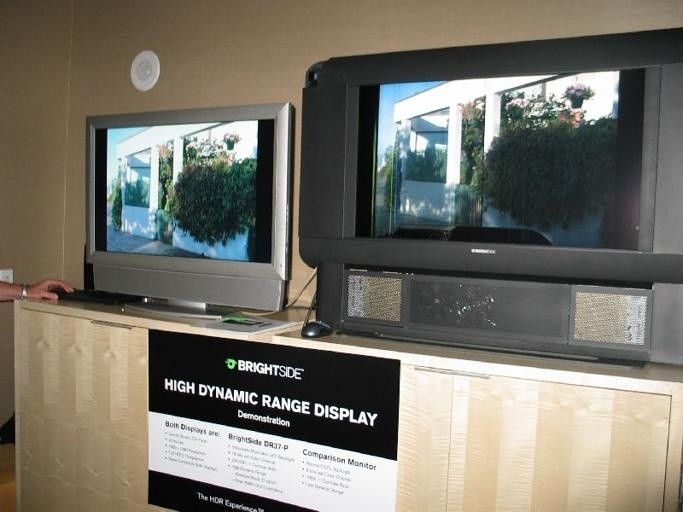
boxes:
[301,319,334,338]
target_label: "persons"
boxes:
[0,279,75,302]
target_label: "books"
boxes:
[192,311,298,337]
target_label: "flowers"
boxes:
[560,84,597,104]
[222,132,241,144]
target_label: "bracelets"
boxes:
[20,283,28,300]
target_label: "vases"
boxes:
[570,98,584,108]
[227,143,234,150]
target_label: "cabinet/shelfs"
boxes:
[12,295,683,512]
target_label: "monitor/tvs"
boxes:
[86,101,292,319]
[300,26,683,366]
